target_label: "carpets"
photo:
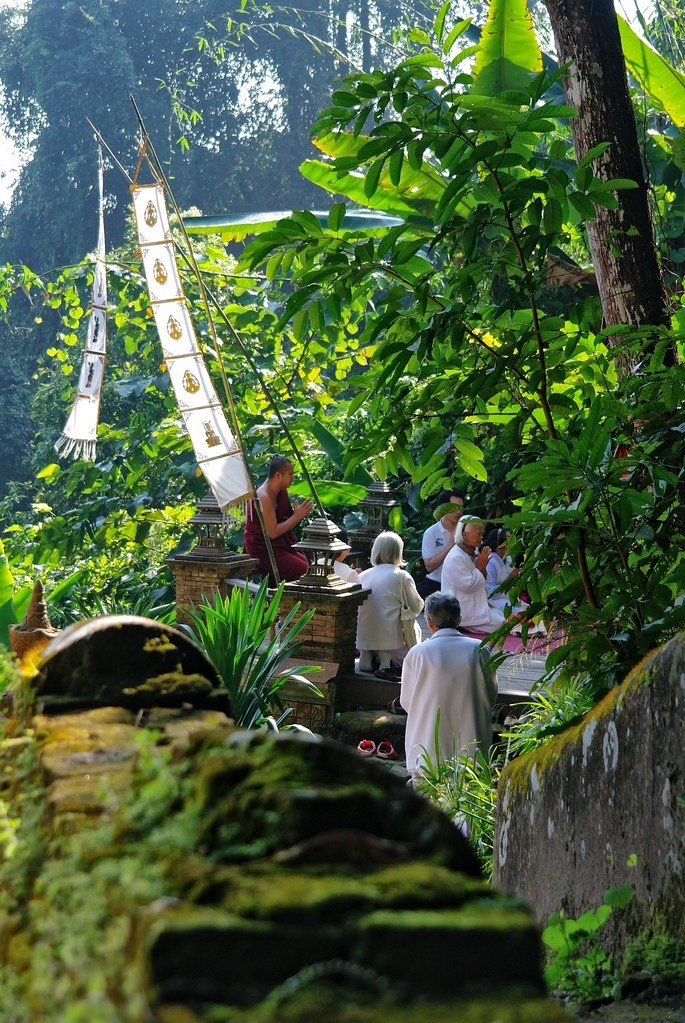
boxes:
[463,626,566,654]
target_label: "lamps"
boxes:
[184,494,236,556]
[356,482,400,533]
[290,517,352,588]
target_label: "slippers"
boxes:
[377,741,402,759]
[386,697,407,715]
[357,739,376,756]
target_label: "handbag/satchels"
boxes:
[400,571,422,648]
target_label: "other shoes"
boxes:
[374,668,401,681]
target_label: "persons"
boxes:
[480,529,529,613]
[418,489,463,599]
[356,532,425,671]
[441,515,504,634]
[311,526,361,583]
[399,591,498,837]
[246,455,313,579]
